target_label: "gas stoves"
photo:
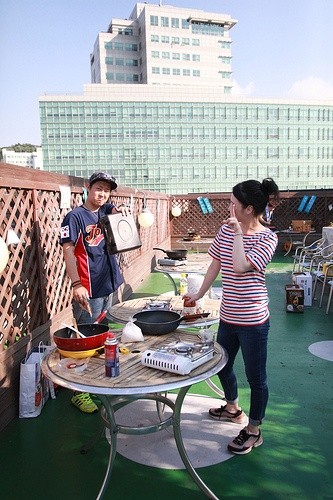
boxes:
[141,336,218,376]
[158,257,187,267]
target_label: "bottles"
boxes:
[184,297,196,322]
[104,333,119,377]
[179,273,188,297]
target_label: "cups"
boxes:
[199,329,215,356]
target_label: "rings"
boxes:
[80,298,83,300]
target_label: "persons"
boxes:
[182,178,279,455]
[259,200,278,227]
[59,172,123,414]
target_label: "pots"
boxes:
[56,346,105,359]
[132,310,211,337]
[153,247,188,259]
[53,310,110,351]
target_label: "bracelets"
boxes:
[72,280,81,287]
[234,234,243,236]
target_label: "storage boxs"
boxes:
[292,272,312,306]
[285,285,304,313]
[292,220,312,231]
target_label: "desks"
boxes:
[155,262,222,299]
[106,295,225,414]
[42,329,228,500]
[281,229,316,256]
[176,238,214,253]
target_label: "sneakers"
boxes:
[226,427,264,455]
[209,404,244,423]
[71,392,99,414]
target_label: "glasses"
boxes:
[91,173,115,180]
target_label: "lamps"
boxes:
[136,192,154,227]
[170,196,181,217]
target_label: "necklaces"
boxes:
[85,204,98,219]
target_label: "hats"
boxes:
[88,172,118,189]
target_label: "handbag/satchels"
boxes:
[17,340,48,418]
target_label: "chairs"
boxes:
[292,227,333,315]
